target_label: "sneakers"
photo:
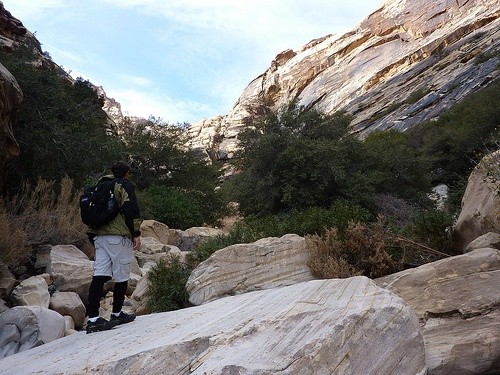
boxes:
[109,311,136,326]
[86,317,114,334]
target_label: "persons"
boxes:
[84,160,142,334]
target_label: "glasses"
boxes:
[127,171,130,175]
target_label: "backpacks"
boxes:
[79,179,120,229]
